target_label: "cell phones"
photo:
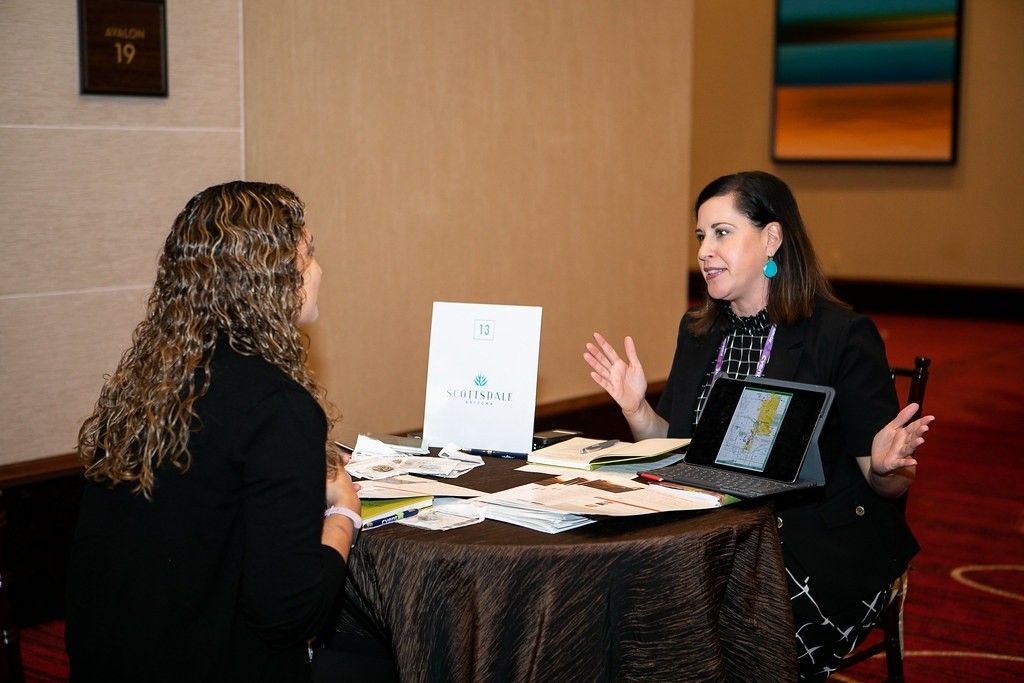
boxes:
[533,430,581,446]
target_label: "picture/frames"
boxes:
[769,0,967,168]
[78,0,169,98]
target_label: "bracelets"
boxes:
[324,504,362,548]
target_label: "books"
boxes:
[355,436,693,532]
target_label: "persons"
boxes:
[582,169,935,683]
[62,180,401,683]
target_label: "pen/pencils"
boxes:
[637,472,663,482]
[363,508,417,529]
[580,440,619,454]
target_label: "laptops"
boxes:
[638,373,836,497]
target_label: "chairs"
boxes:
[0,474,83,683]
[831,354,932,683]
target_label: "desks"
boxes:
[348,432,800,683]
